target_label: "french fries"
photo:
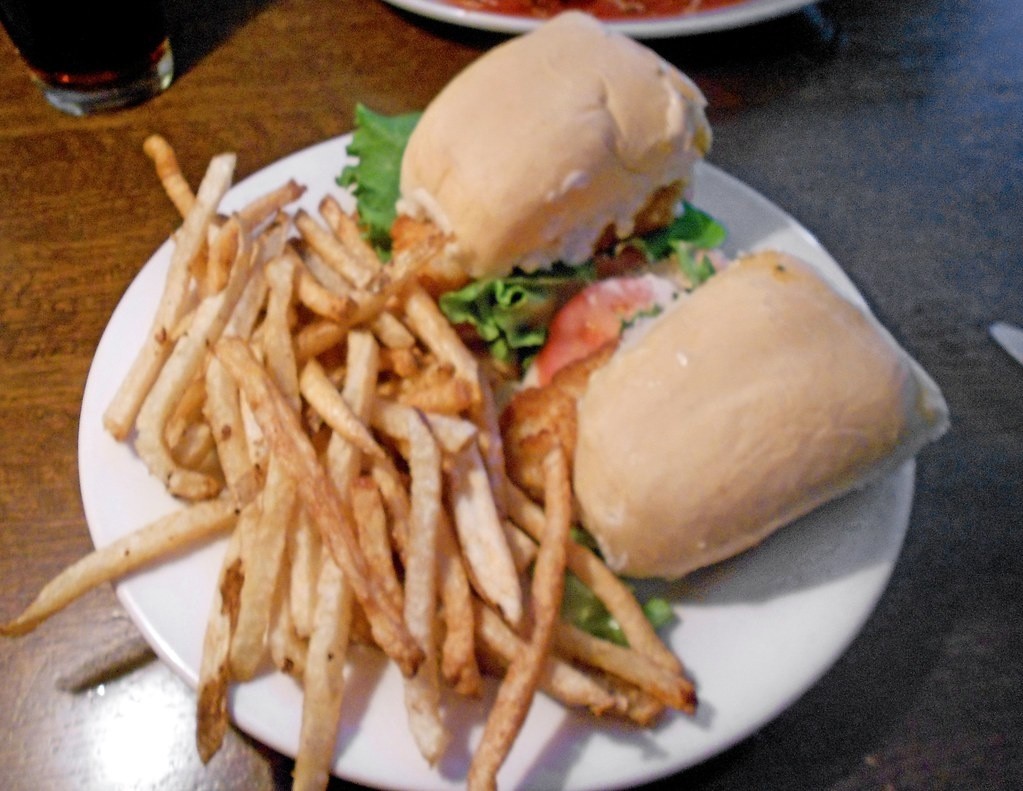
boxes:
[0,132,698,790]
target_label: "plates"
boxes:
[386,1,821,39]
[76,128,914,791]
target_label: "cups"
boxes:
[0,0,175,120]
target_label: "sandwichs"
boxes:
[498,234,964,638]
[340,11,720,364]
